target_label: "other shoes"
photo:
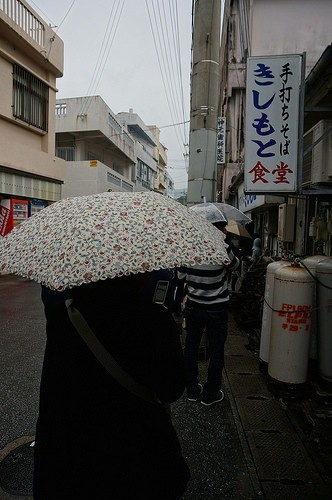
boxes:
[188,383,203,403]
[201,390,225,405]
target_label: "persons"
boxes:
[32,270,191,500]
[175,211,242,406]
[230,222,262,291]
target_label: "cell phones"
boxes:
[153,281,169,304]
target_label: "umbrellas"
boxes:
[190,197,252,224]
[0,192,232,291]
[225,218,252,240]
